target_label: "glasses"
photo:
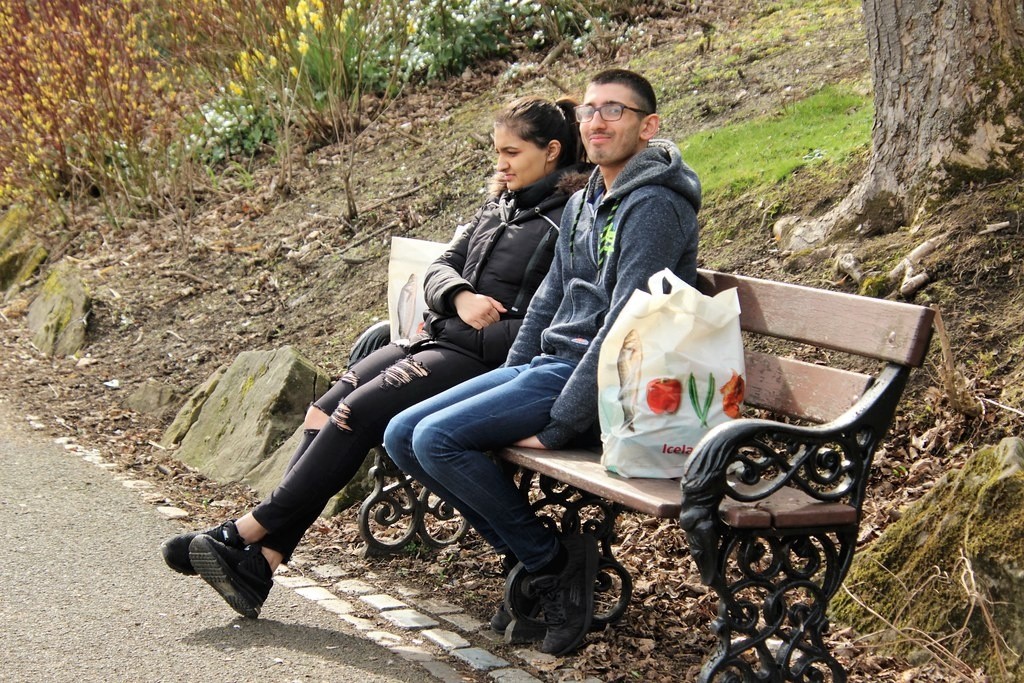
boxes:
[573,103,650,123]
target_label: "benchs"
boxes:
[356,246,942,683]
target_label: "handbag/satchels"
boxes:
[385,224,466,346]
[597,268,746,480]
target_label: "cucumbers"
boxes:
[688,371,716,428]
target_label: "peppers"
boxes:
[647,378,682,415]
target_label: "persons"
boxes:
[162,94,596,618]
[383,66,704,656]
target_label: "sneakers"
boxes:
[162,518,248,576]
[492,557,545,635]
[529,534,599,656]
[189,534,275,619]
